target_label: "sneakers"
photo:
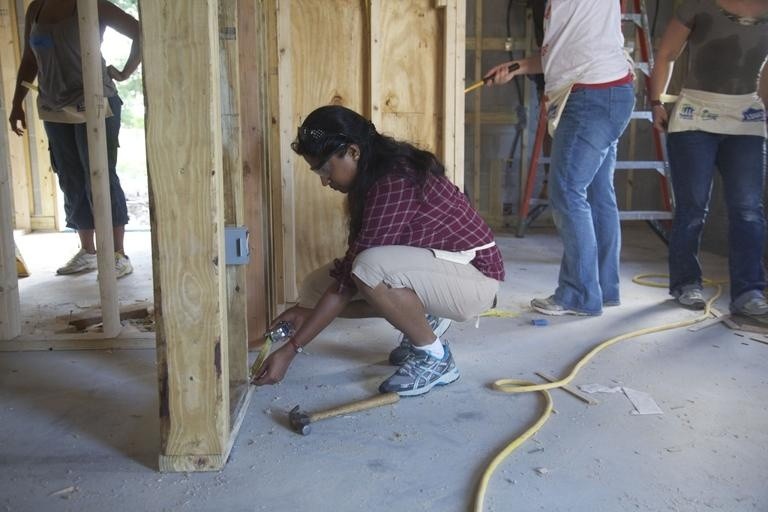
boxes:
[389,312,451,364]
[673,287,705,308]
[57,247,97,275]
[531,294,594,317]
[113,252,133,279]
[379,339,460,398]
[734,296,768,326]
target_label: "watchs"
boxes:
[289,337,303,355]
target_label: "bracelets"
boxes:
[651,98,663,107]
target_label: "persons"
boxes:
[253,104,504,399]
[487,0,634,314]
[646,1,768,317]
[9,2,141,283]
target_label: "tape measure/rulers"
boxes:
[250,320,288,379]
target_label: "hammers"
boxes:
[289,393,400,436]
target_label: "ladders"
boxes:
[516,0,676,248]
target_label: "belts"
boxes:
[572,73,632,90]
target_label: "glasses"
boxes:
[309,144,342,180]
